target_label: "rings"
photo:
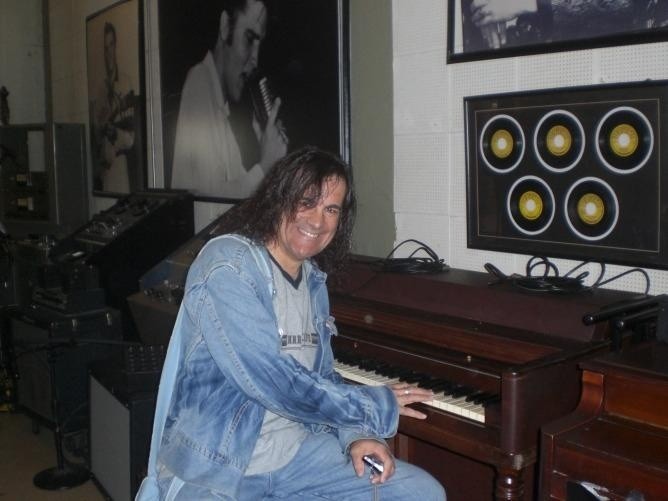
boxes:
[404,388,411,395]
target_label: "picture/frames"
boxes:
[445,1,668,67]
[145,1,352,215]
[86,1,147,198]
[462,79,667,271]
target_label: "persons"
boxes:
[462,0,539,50]
[92,20,137,193]
[170,0,290,234]
[135,145,448,501]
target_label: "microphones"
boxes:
[248,73,282,125]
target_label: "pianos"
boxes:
[535,304,667,501]
[314,252,660,501]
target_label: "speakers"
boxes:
[87,361,163,501]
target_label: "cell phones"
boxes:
[363,454,384,472]
